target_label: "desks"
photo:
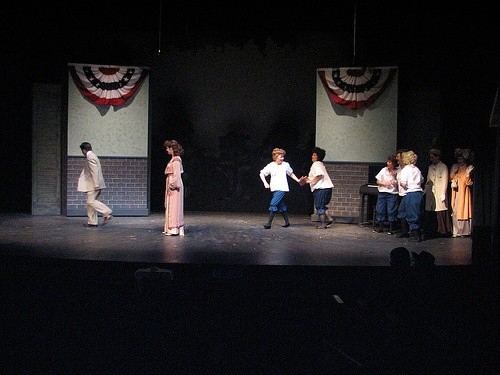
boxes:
[359,184,379,227]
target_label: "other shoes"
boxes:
[461,235,466,238]
[263,225,271,229]
[165,234,172,236]
[325,218,334,228]
[101,214,113,226]
[83,223,97,228]
[282,223,290,227]
[316,225,325,229]
[162,232,166,233]
[452,235,457,237]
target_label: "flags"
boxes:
[318,68,393,110]
[69,65,152,106]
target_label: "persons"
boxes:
[389,246,439,290]
[449,148,476,238]
[423,149,452,237]
[372,155,399,235]
[399,151,426,242]
[162,140,185,237]
[305,147,335,229]
[389,149,414,238]
[77,141,113,229]
[259,148,307,229]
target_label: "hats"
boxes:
[411,251,435,267]
[428,149,441,156]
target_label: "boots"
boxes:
[394,218,409,238]
[388,221,394,235]
[373,221,383,234]
[411,229,421,242]
